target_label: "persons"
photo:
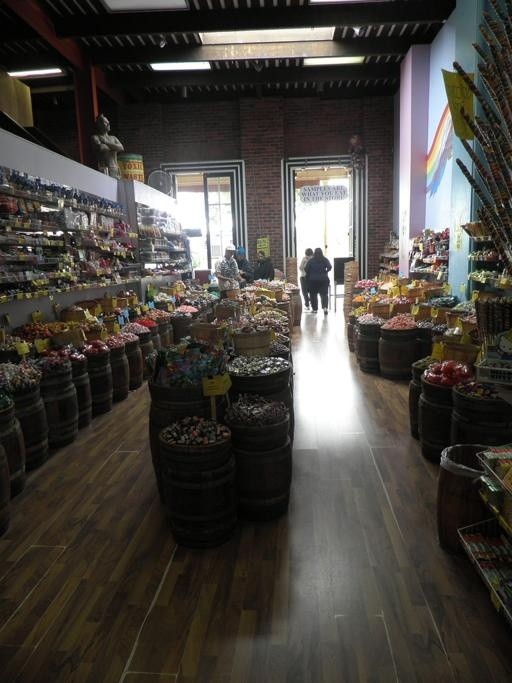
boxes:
[216,245,275,298]
[90,114,123,178]
[300,248,331,314]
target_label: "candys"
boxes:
[153,276,292,448]
[347,221,512,406]
[0,165,217,419]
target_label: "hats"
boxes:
[226,243,236,251]
[235,246,246,256]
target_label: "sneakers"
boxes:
[305,304,328,315]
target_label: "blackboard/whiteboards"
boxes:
[334,257,355,284]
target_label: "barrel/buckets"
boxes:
[0,280,302,549]
[344,281,512,553]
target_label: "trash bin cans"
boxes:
[436,444,497,550]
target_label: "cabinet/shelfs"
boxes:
[378,233,511,304]
[0,164,194,304]
